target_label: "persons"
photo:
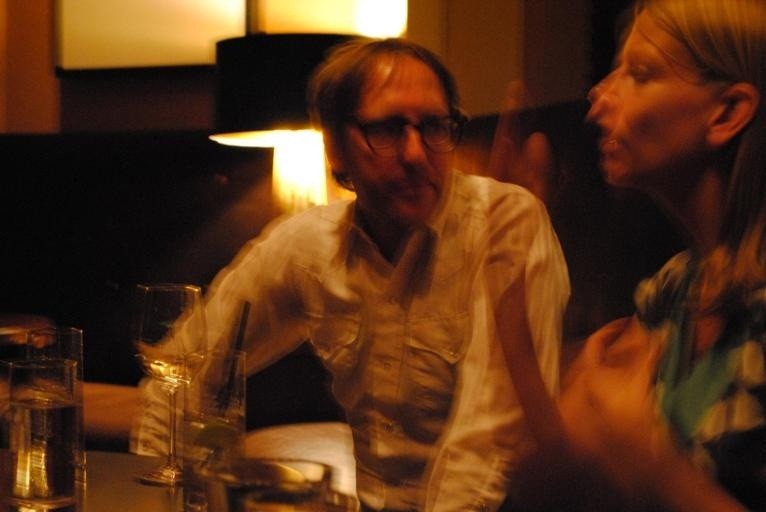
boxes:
[129,37,574,512]
[483,2,766,511]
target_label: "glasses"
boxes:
[342,104,475,156]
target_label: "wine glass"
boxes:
[126,284,208,487]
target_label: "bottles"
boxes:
[180,351,362,511]
[1,328,91,512]
